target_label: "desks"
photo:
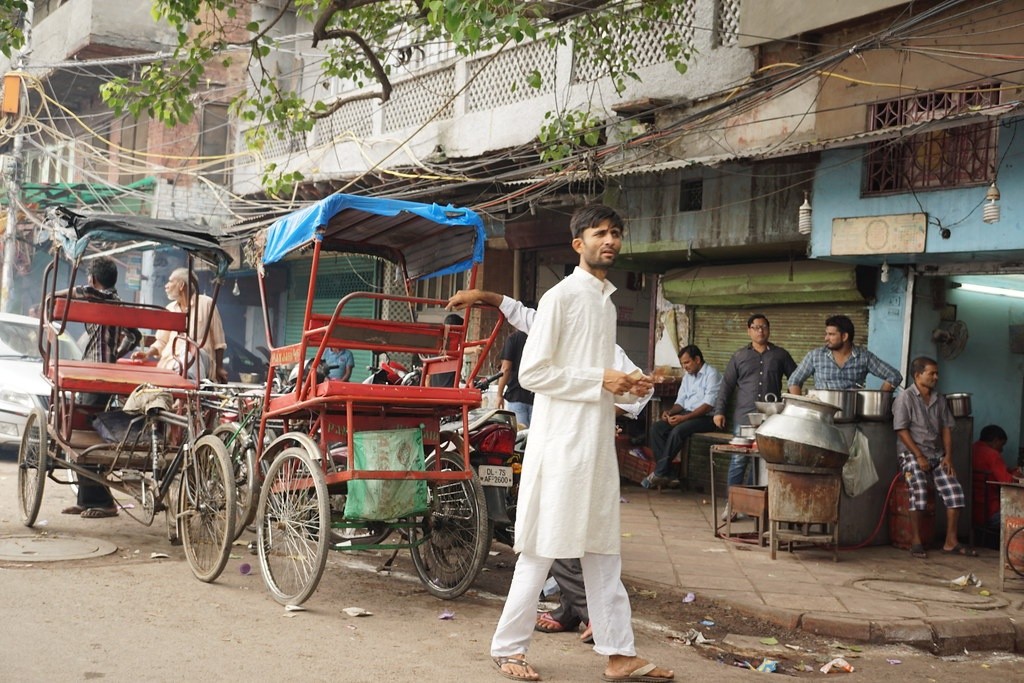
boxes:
[626,397,676,447]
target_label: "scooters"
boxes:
[177,364,529,588]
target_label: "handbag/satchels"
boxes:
[841,428,879,497]
[92,399,164,443]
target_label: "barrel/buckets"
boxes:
[943,392,973,417]
[806,387,893,422]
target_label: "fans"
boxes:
[933,320,970,362]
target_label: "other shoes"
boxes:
[667,475,679,487]
[721,503,737,521]
[641,472,669,488]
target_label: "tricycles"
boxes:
[17,209,237,584]
[195,193,498,608]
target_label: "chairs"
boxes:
[972,468,1000,548]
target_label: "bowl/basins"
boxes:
[240,373,260,383]
[728,401,785,446]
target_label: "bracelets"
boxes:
[915,455,925,459]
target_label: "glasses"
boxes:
[749,324,768,331]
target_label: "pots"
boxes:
[755,393,850,468]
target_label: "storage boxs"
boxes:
[619,449,678,484]
[651,382,681,396]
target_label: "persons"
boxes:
[714,313,805,521]
[642,344,722,490]
[495,298,536,427]
[47,256,142,519]
[891,355,982,558]
[321,346,355,382]
[489,204,677,683]
[974,425,1019,544]
[787,315,903,395]
[130,266,229,383]
[424,314,465,386]
[445,289,655,644]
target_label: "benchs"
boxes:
[686,433,734,445]
[270,293,505,411]
[41,294,200,398]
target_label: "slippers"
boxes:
[490,656,539,680]
[941,543,978,556]
[534,611,580,632]
[61,504,86,513]
[581,619,593,642]
[601,664,672,682]
[910,544,928,558]
[81,507,119,517]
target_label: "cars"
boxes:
[0,311,84,460]
